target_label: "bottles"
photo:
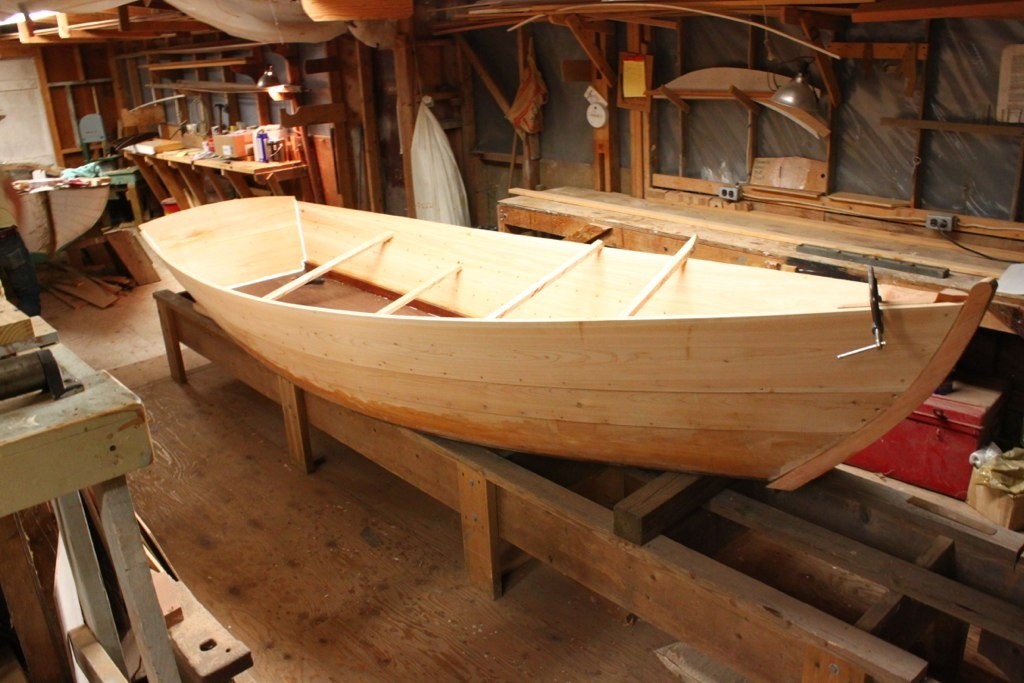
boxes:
[256,129,270,163]
[208,136,215,153]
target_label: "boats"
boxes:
[136,196,998,492]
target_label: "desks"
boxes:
[498,187,1024,337]
[123,140,321,212]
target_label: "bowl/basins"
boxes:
[160,197,180,213]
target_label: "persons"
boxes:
[0,169,41,318]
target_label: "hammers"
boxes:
[214,103,225,135]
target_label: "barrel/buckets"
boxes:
[268,139,286,163]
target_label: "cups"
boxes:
[186,123,198,133]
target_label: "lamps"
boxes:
[766,56,818,111]
[256,64,280,89]
